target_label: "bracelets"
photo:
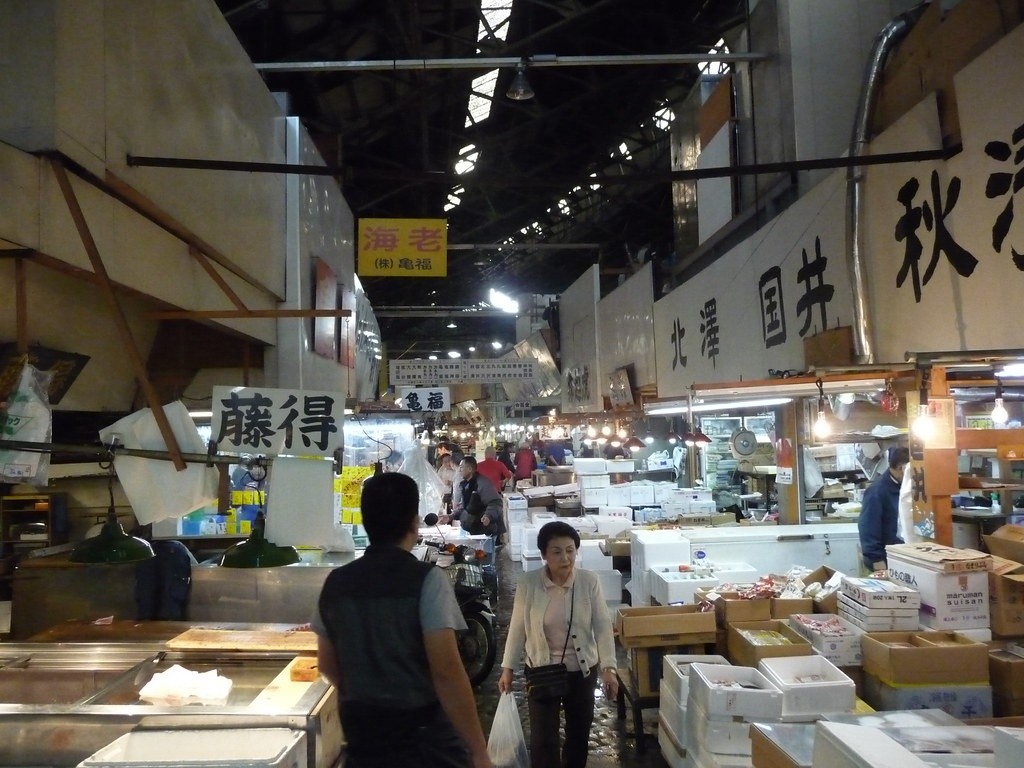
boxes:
[602,667,617,676]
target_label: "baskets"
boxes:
[439,562,484,593]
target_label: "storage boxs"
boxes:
[150,489,264,535]
[333,430,1024,768]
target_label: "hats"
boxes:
[887,446,909,462]
[504,442,515,447]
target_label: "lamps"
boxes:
[827,393,856,422]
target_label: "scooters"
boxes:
[423,512,498,688]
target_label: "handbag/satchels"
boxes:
[524,662,572,705]
[485,691,531,768]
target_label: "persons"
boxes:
[856,446,906,573]
[438,456,506,546]
[312,473,497,767]
[498,521,620,768]
[383,434,632,513]
